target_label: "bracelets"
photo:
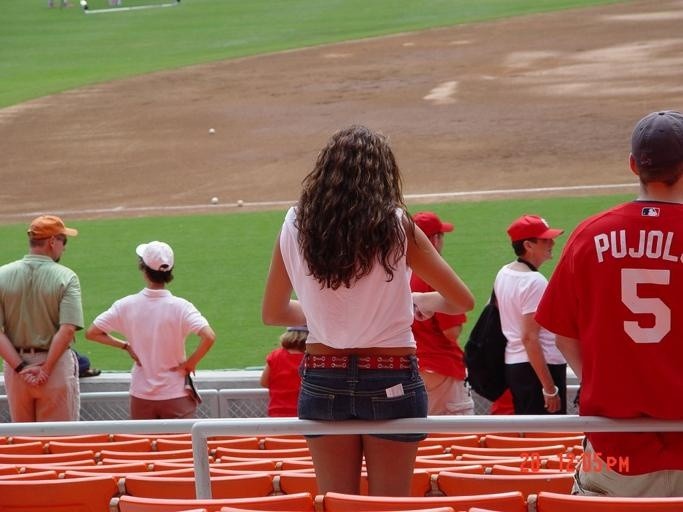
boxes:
[15,361,29,373]
[542,385,559,397]
[122,341,128,349]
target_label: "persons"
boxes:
[409,211,476,416]
[260,324,310,417]
[0,215,84,422]
[263,124,475,496]
[72,348,101,377]
[86,240,216,420]
[486,215,567,414]
[534,110,683,497]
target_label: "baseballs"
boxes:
[213,197,218,202]
[210,128,216,133]
[238,200,243,206]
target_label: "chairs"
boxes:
[1,426,683,511]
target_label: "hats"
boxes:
[508,215,563,242]
[27,217,77,239]
[136,240,174,273]
[631,111,683,167]
[411,213,453,236]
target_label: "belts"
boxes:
[302,352,420,371]
[14,347,49,353]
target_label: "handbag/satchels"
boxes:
[463,284,509,402]
